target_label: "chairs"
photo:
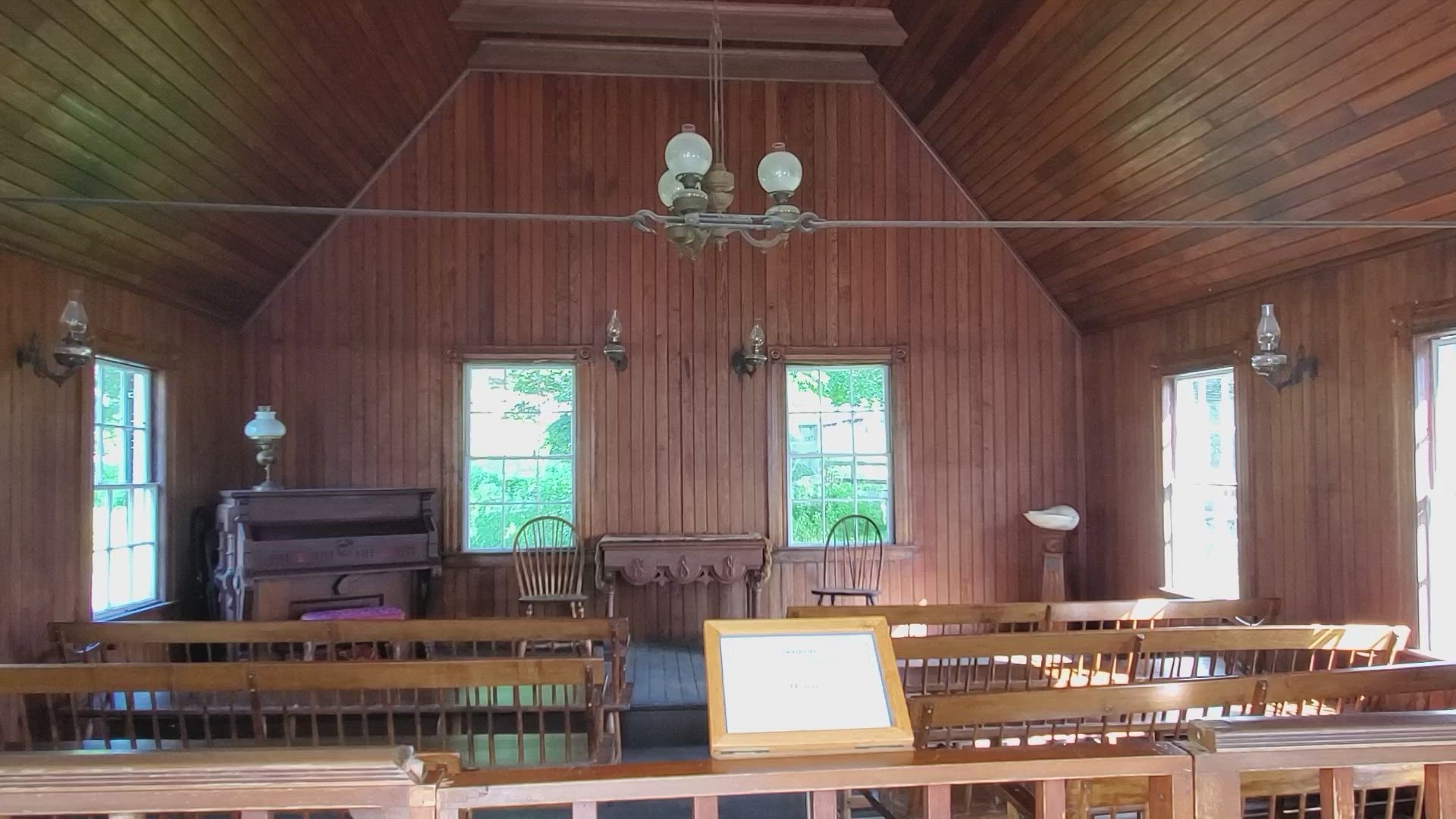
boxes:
[512,514,593,658]
[812,514,883,605]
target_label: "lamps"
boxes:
[1251,305,1316,390]
[654,126,803,259]
[603,309,627,372]
[734,324,768,377]
[245,406,285,490]
[15,296,94,388]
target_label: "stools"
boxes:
[301,606,406,660]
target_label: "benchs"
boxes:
[0,617,633,819]
[791,596,1427,819]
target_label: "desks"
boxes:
[596,533,773,619]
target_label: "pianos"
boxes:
[204,486,449,669]
